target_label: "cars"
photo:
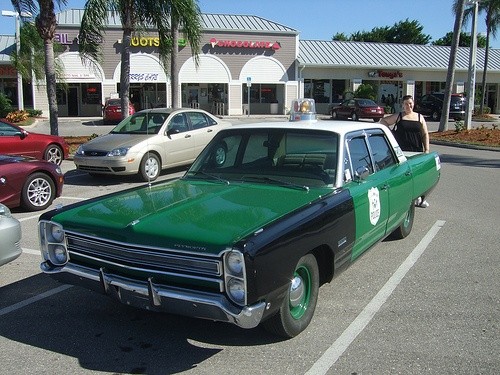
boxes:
[0,202,24,266]
[0,117,69,166]
[73,106,238,182]
[100,98,136,124]
[0,153,64,212]
[38,98,441,339]
[331,99,384,122]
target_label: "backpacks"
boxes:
[387,97,391,106]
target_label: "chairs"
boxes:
[252,151,371,184]
[143,116,205,136]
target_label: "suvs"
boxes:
[413,92,468,121]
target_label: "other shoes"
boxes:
[414,197,429,207]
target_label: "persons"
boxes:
[380,95,430,209]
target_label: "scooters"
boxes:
[327,103,334,115]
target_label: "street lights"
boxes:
[1,10,33,110]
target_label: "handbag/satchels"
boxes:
[390,114,400,135]
